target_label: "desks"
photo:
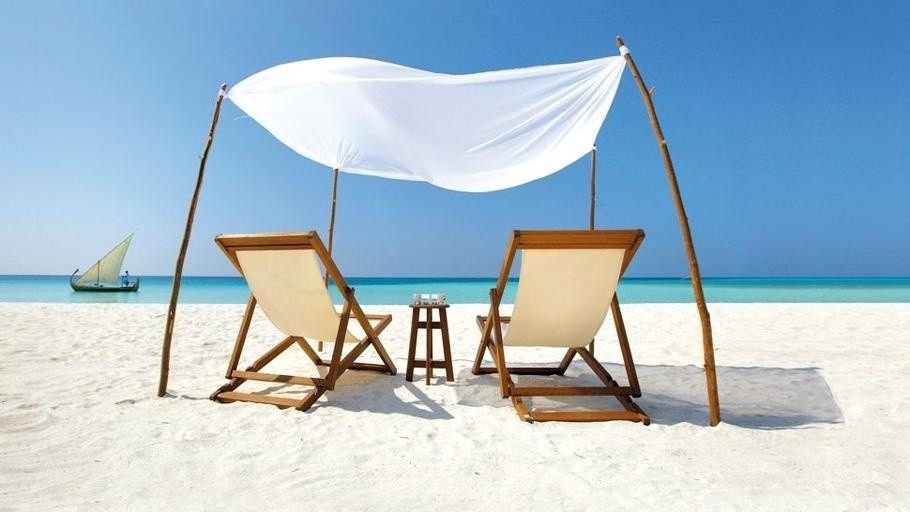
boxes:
[405,304,454,386]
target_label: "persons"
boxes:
[124,271,130,287]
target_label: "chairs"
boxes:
[210,230,398,410]
[472,229,650,425]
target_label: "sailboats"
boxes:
[70,231,140,292]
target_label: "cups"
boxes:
[413,293,445,305]
